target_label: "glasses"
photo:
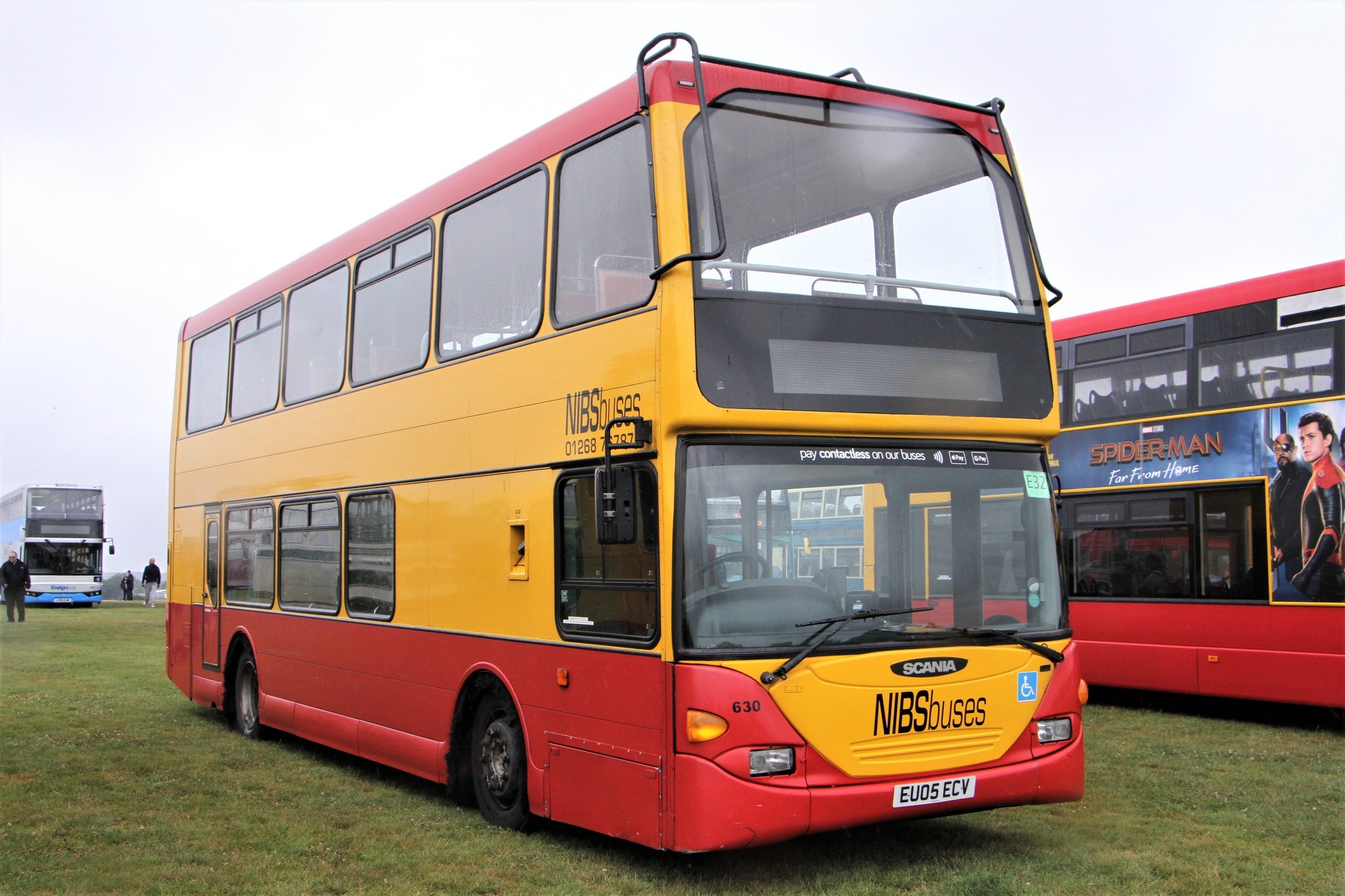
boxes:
[9,557,16,558]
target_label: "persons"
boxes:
[1110,554,1169,596]
[1223,565,1230,589]
[0,551,31,622]
[121,570,134,601]
[1269,411,1345,602]
[142,558,161,607]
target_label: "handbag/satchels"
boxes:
[121,583,124,589]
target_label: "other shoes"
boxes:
[9,619,15,622]
[19,619,25,622]
[122,600,124,602]
[143,602,148,607]
[150,604,155,608]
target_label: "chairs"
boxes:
[1075,376,1305,422]
[1077,579,1230,599]
[32,506,96,519]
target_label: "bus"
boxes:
[0,482,115,608]
[705,482,865,612]
[165,27,1087,855]
[869,255,1345,711]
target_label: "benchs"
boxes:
[202,252,921,429]
